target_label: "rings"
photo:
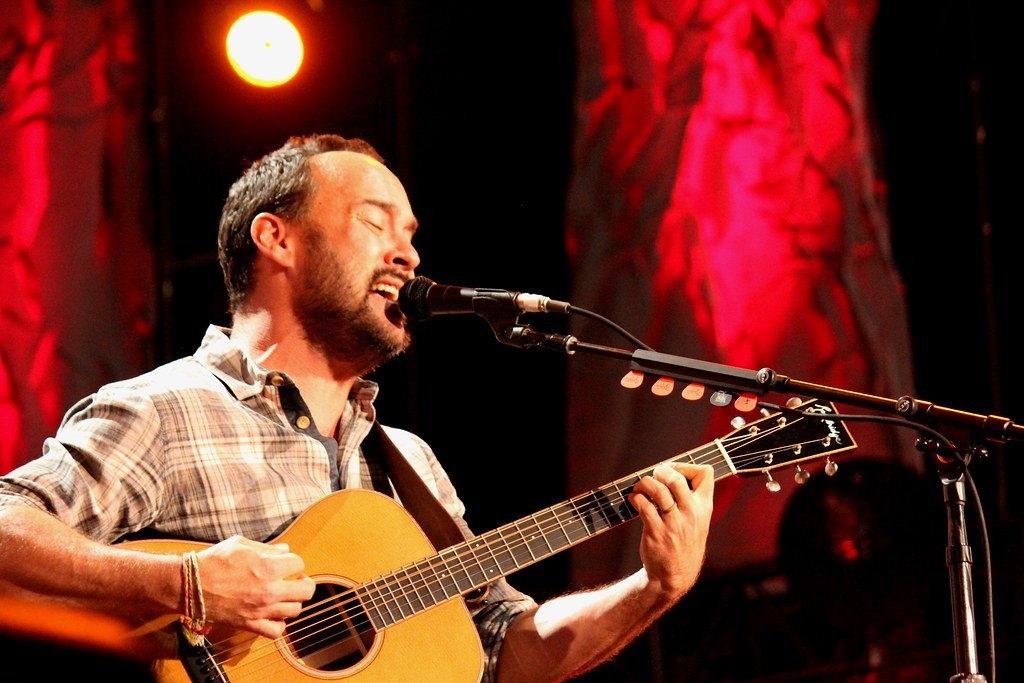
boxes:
[660,502,677,514]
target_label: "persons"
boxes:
[0,136,714,683]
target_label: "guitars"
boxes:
[111,397,858,682]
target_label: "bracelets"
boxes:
[180,550,212,646]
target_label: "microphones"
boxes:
[399,276,570,322]
